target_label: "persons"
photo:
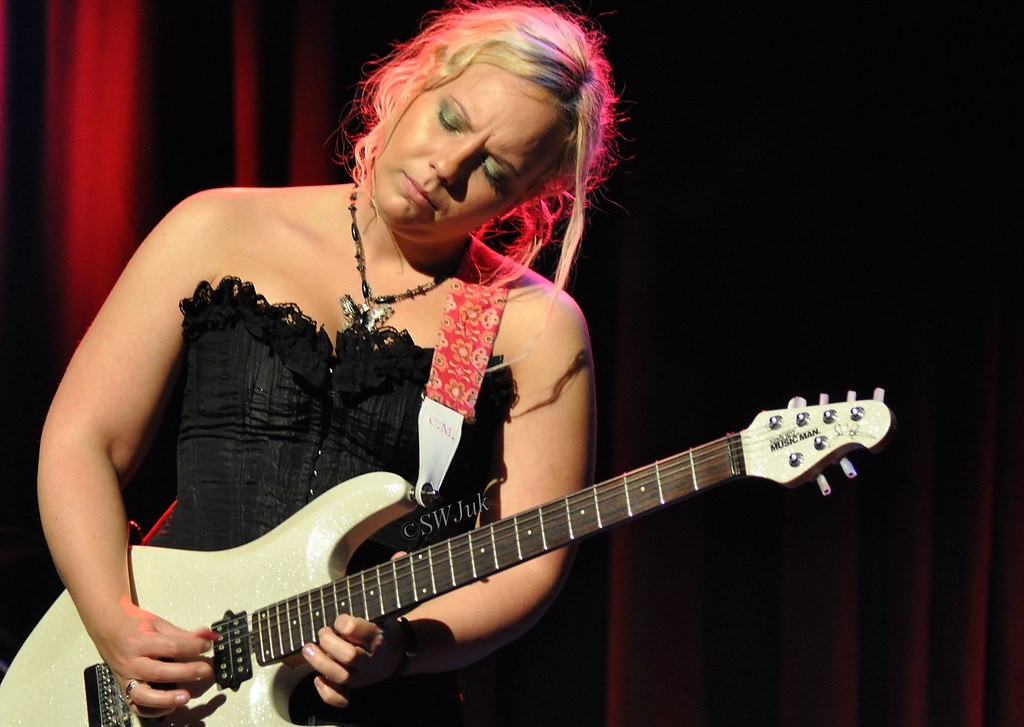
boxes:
[37,1,616,727]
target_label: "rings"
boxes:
[125,678,143,700]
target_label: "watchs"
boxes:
[391,617,417,678]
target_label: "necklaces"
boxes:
[340,183,472,333]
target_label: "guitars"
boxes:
[2,385,895,726]
